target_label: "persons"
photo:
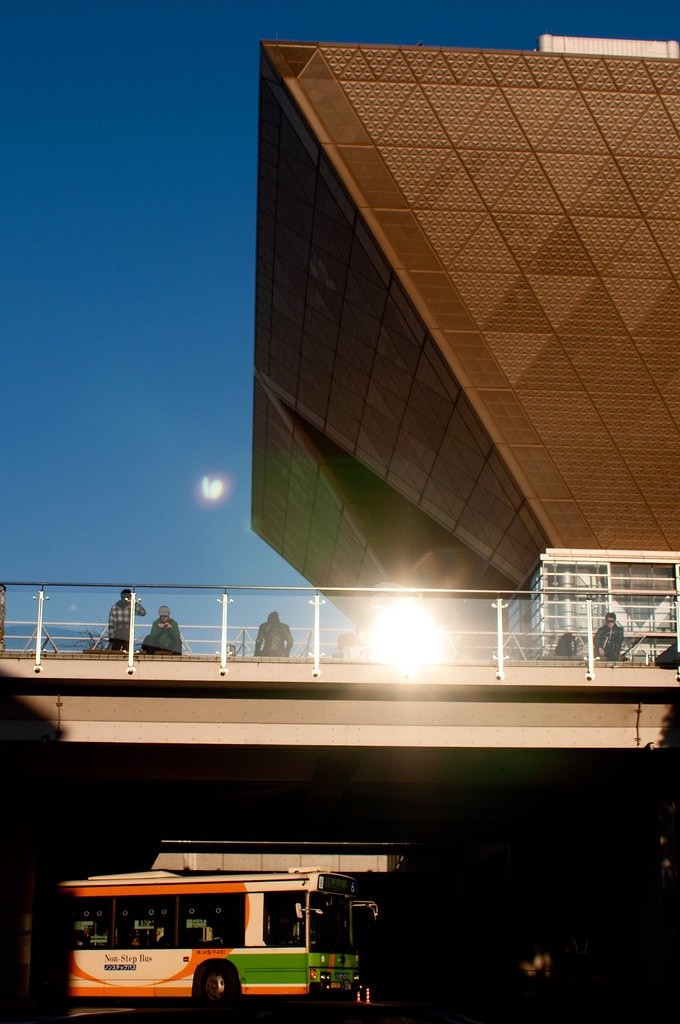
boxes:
[252,610,294,657]
[589,612,625,661]
[140,605,185,655]
[107,586,146,653]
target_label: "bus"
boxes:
[57,868,382,1013]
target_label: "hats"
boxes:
[159,606,170,615]
